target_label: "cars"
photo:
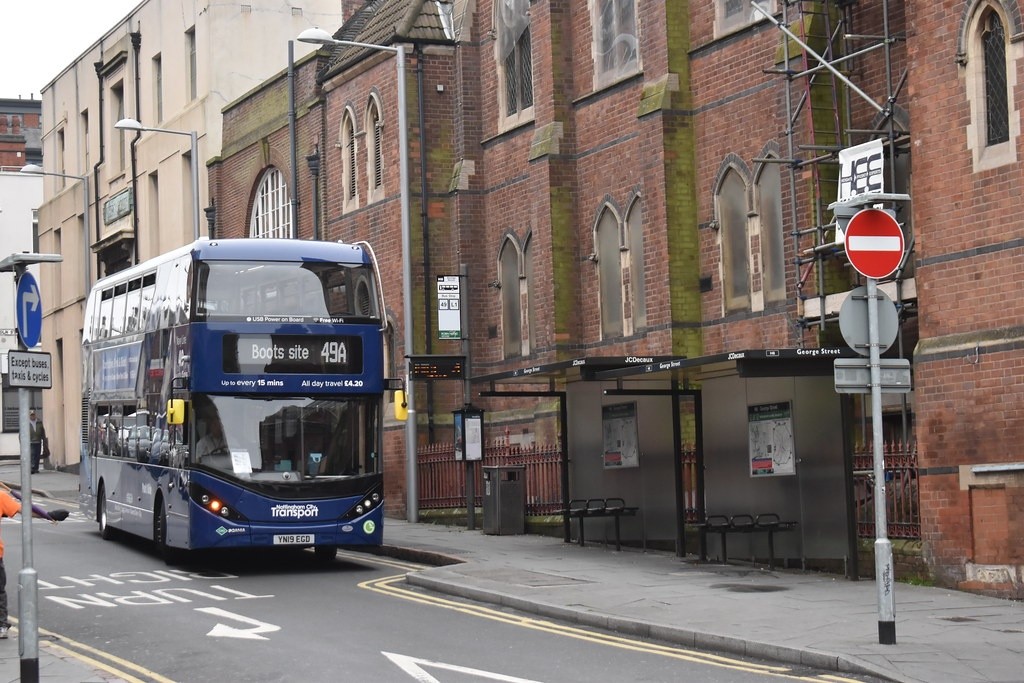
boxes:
[101,411,182,456]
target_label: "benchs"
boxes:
[687,512,800,570]
[551,497,639,551]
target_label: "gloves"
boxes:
[47,510,69,521]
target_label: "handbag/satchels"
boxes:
[40,439,50,459]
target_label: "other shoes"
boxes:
[31,470,39,474]
[0,627,8,639]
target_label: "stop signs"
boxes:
[844,207,905,279]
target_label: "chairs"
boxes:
[197,417,213,444]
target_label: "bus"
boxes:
[78,236,406,559]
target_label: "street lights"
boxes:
[113,118,200,243]
[296,26,418,523]
[20,164,91,305]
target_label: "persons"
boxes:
[0,481,70,639]
[196,415,241,463]
[19,407,46,474]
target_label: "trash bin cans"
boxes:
[480,465,527,534]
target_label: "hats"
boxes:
[30,410,35,415]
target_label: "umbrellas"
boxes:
[0,481,59,524]
